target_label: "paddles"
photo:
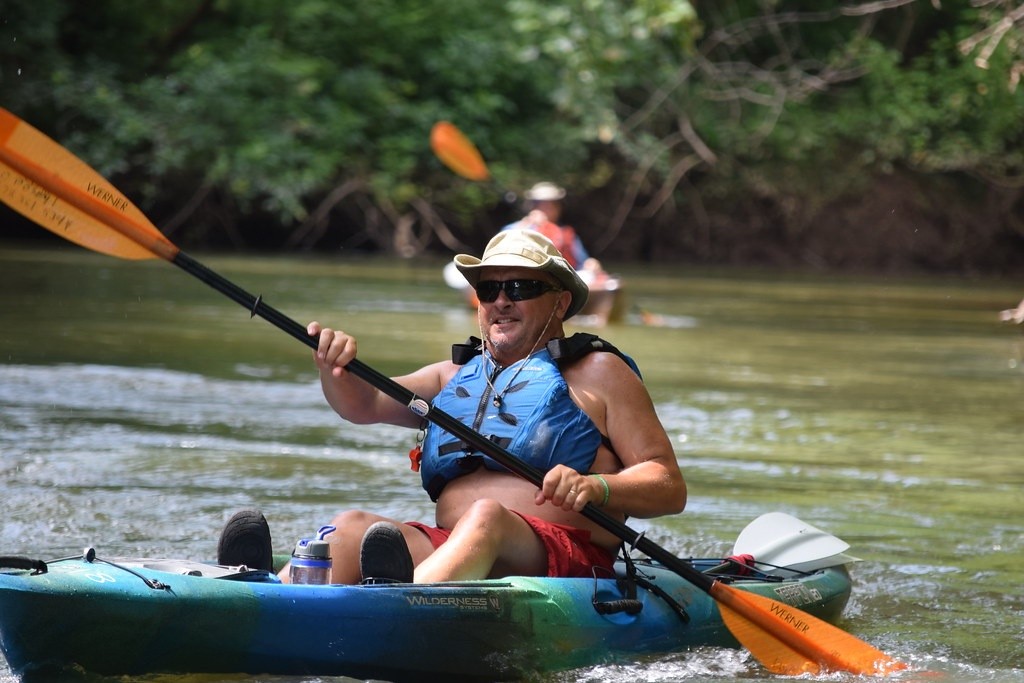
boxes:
[0,107,941,683]
[430,120,654,321]
[702,510,864,583]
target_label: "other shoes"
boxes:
[359,521,414,585]
[216,508,274,574]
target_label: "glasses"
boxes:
[476,278,563,303]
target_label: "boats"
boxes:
[0,547,852,683]
[465,276,624,324]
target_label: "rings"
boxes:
[570,490,578,495]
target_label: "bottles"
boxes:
[290,526,336,586]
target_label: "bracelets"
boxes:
[594,475,609,506]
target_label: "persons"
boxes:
[217,229,687,585]
[502,183,600,275]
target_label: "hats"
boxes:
[454,228,590,322]
[523,181,566,202]
